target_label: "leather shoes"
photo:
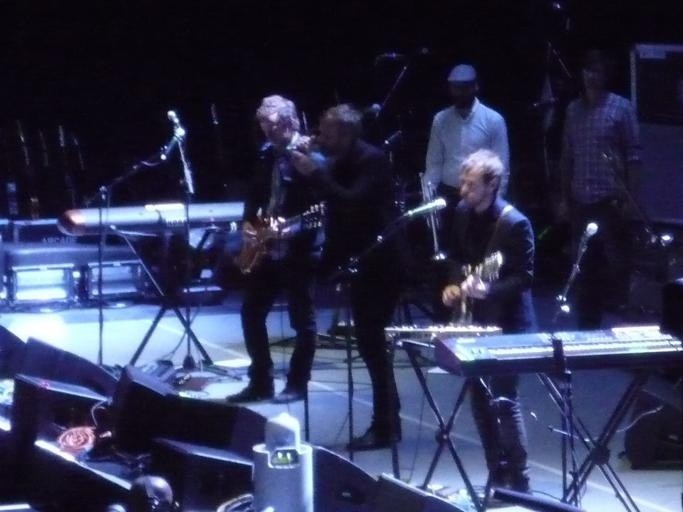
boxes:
[346,417,401,450]
[272,392,303,403]
[226,383,274,402]
[484,471,530,508]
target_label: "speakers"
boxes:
[151,436,257,507]
[11,372,109,441]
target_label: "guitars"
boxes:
[235,202,325,276]
[450,251,503,326]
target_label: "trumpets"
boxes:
[419,172,447,263]
[600,152,674,249]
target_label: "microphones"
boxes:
[168,110,180,129]
[577,222,598,255]
[408,198,447,219]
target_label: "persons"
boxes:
[292,102,408,452]
[441,147,537,507]
[422,64,511,327]
[558,58,643,329]
[227,95,327,405]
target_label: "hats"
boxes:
[447,64,477,86]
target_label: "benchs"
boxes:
[0,215,150,308]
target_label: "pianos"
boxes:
[56,200,246,237]
[435,325,683,376]
[384,325,504,344]
